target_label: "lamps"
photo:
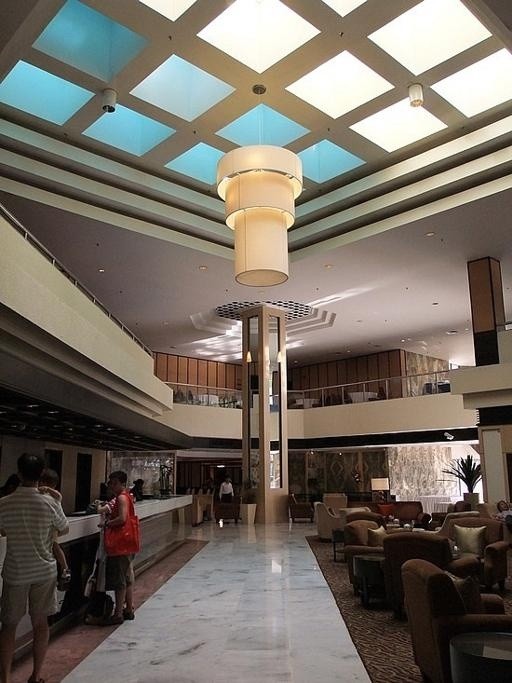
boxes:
[212,85,304,290]
[407,83,426,108]
[101,88,118,114]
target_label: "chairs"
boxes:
[314,491,512,681]
[287,386,386,409]
[185,486,217,527]
[288,491,314,523]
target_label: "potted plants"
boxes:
[441,454,484,506]
[238,478,260,527]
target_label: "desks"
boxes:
[198,394,219,406]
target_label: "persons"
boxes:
[0,473,20,499]
[0,451,69,682]
[39,466,73,590]
[414,514,431,530]
[218,475,234,504]
[411,512,424,527]
[496,500,512,534]
[95,469,146,625]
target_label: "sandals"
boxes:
[60,566,72,584]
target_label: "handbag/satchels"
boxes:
[84,591,114,625]
[103,515,141,556]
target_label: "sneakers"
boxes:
[123,608,134,619]
[110,614,124,623]
